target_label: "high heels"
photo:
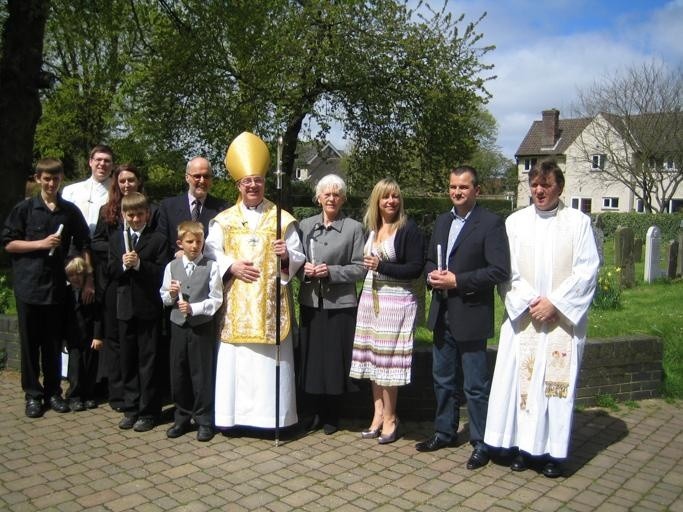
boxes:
[362,416,401,443]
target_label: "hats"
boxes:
[225,131,271,181]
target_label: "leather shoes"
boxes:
[512,452,563,478]
[26,396,96,418]
[167,424,213,441]
[415,436,456,451]
[117,416,154,431]
[467,449,489,469]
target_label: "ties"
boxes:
[192,200,201,220]
[187,263,194,277]
[131,234,138,249]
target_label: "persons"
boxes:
[1,130,602,479]
